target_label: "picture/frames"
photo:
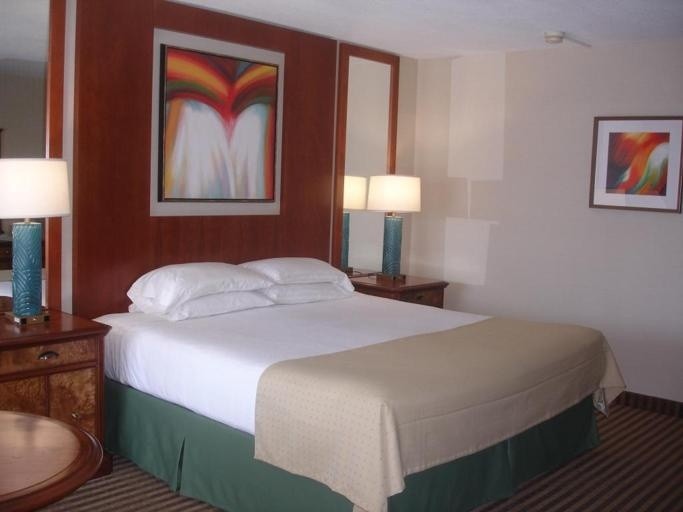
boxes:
[588,116,682,213]
[152,29,286,221]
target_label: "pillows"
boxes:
[126,257,357,323]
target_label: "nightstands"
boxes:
[350,272,449,308]
[0,309,113,449]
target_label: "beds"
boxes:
[93,291,627,511]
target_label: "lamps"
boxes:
[339,176,367,273]
[0,157,70,324]
[367,175,421,283]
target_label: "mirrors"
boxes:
[332,42,401,276]
[0,0,67,319]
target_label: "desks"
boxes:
[0,409,104,512]
